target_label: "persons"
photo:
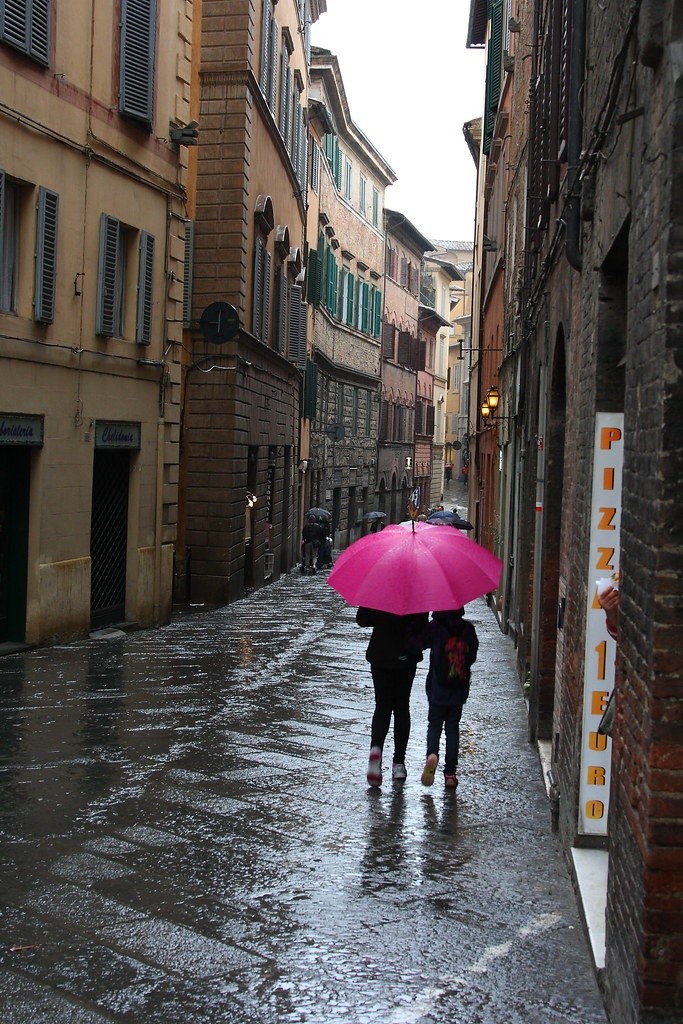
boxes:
[355,604,432,787]
[421,605,479,788]
[428,506,444,513]
[301,515,333,571]
[416,511,427,523]
[452,508,461,520]
[597,584,620,644]
[370,518,378,534]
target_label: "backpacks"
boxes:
[436,617,473,690]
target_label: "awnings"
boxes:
[382,207,439,251]
[419,304,455,328]
[424,256,466,281]
[308,98,337,135]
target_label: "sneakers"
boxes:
[444,773,458,787]
[393,763,407,778]
[367,746,382,787]
[421,754,438,786]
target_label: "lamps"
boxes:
[481,400,490,420]
[486,385,501,418]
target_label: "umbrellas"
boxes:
[424,511,475,531]
[305,508,329,520]
[325,515,504,615]
[362,512,386,519]
[450,504,468,511]
[433,502,445,507]
